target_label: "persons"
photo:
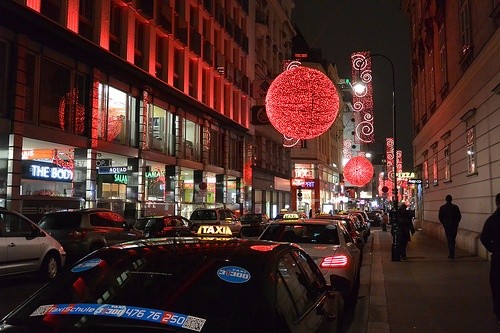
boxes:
[438,195,462,260]
[390,203,416,260]
[479,193,500,324]
[329,210,332,216]
[309,209,312,218]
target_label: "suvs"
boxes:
[38,208,143,261]
[371,210,384,223]
[189,207,243,238]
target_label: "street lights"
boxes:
[381,165,384,176]
[354,54,400,262]
[365,151,388,231]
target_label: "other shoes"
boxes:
[448,255,455,259]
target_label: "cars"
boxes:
[341,211,370,242]
[367,213,381,226]
[311,215,361,251]
[275,214,299,220]
[134,216,197,238]
[0,207,67,278]
[0,237,343,333]
[240,213,270,233]
[258,220,360,299]
